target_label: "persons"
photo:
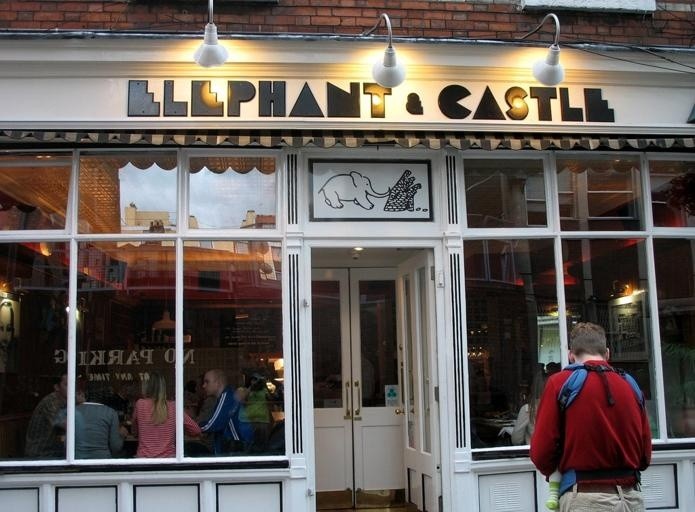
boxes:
[21,367,285,460]
[546,470,563,511]
[529,321,652,512]
[467,358,695,448]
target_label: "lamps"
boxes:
[514,12,565,89]
[362,10,411,89]
[193,0,230,70]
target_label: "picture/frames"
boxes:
[604,290,649,363]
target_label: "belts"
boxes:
[565,482,636,494]
